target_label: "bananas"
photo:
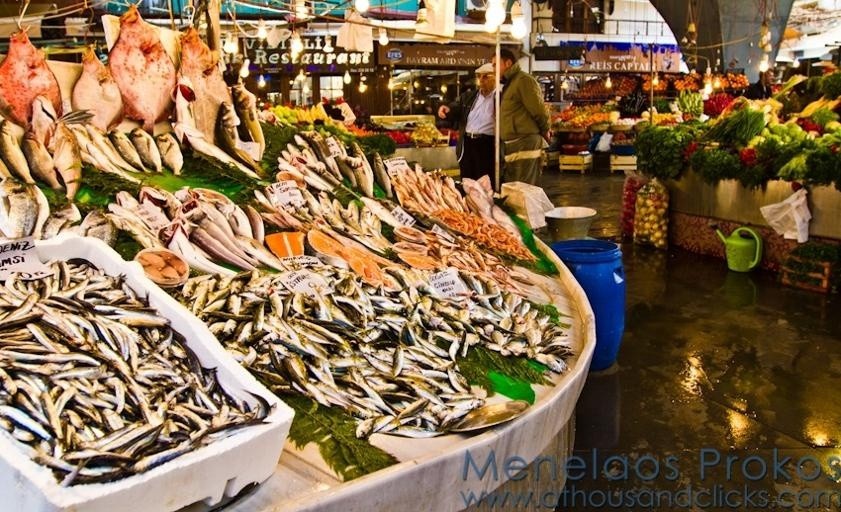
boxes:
[674,88,703,116]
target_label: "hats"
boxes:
[474,62,495,74]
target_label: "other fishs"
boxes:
[0,4,267,201]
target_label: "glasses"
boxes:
[476,74,494,79]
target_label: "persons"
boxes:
[492,48,551,185]
[438,63,503,192]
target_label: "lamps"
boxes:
[220,0,530,92]
[605,74,613,89]
[560,73,571,90]
[652,68,659,85]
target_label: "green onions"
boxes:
[724,102,772,141]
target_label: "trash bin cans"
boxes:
[552,236,628,374]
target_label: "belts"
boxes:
[465,131,494,139]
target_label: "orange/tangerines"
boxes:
[633,185,669,248]
